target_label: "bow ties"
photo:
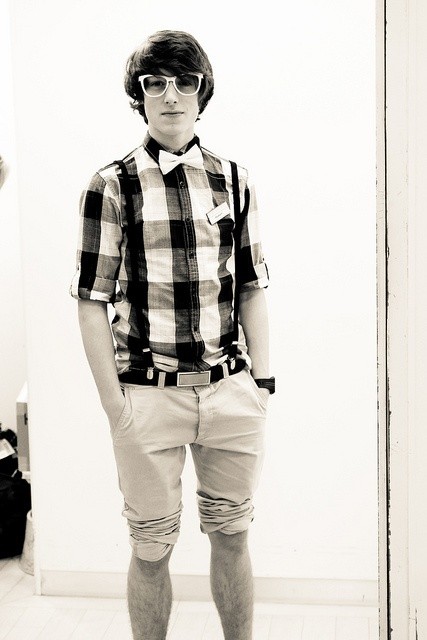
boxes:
[158,145,205,175]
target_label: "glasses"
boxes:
[138,72,204,97]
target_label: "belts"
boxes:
[119,357,246,387]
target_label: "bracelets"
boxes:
[254,376,274,394]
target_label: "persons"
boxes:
[68,31,275,640]
